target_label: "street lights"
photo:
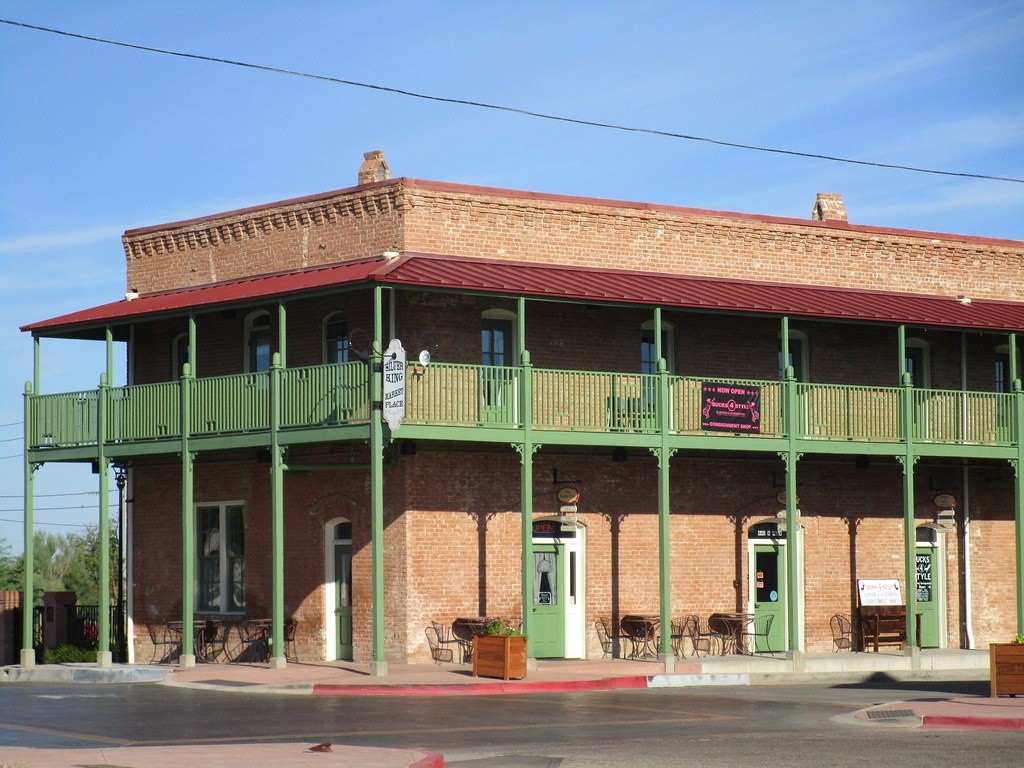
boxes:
[110,462,129,662]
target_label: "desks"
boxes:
[711,613,756,657]
[249,618,288,661]
[458,617,486,663]
[167,620,221,663]
[625,619,660,660]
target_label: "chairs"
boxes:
[235,621,264,664]
[203,622,234,662]
[284,622,299,662]
[691,616,723,658]
[146,623,180,665]
[835,613,870,654]
[656,617,689,659]
[742,615,774,656]
[431,620,472,664]
[600,617,634,660]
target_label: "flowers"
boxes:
[479,616,528,642]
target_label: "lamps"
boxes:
[346,328,396,364]
[405,331,438,369]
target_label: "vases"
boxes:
[473,633,528,681]
